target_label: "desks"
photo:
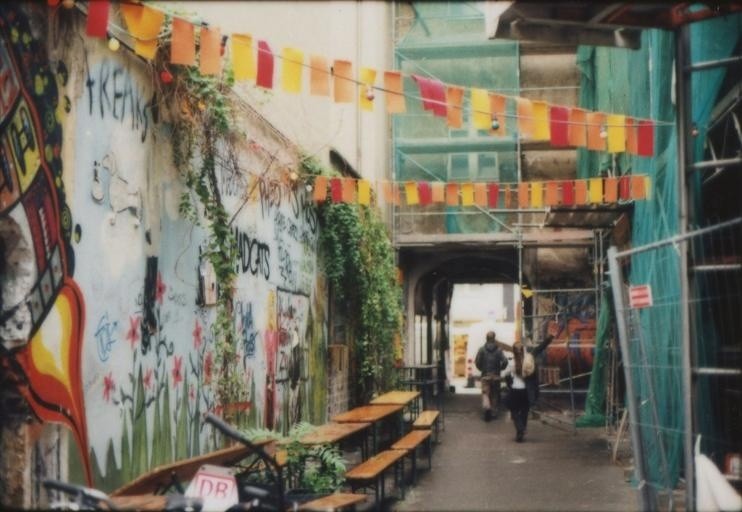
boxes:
[97,436,274,511]
[243,389,422,493]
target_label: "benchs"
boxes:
[284,409,441,511]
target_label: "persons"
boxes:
[499,342,530,443]
[474,331,509,421]
[499,356,514,390]
[521,330,557,408]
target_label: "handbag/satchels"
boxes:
[521,345,536,379]
